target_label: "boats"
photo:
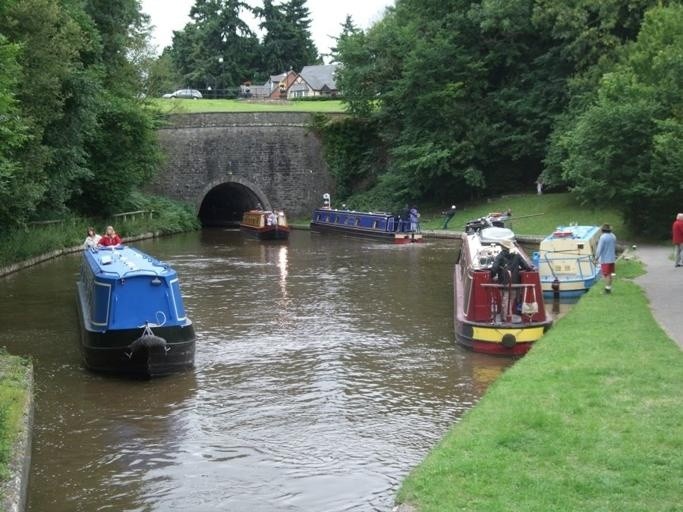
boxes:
[74,242,195,380]
[239,207,288,242]
[452,213,552,357]
[310,193,423,244]
[531,220,603,299]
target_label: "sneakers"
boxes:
[604,287,611,293]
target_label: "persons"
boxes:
[97,226,122,247]
[440,205,456,229]
[593,223,617,294]
[488,236,535,322]
[397,202,419,232]
[671,212,683,267]
[534,178,544,195]
[268,208,278,225]
[84,225,101,250]
[255,201,263,211]
[410,204,418,231]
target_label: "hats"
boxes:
[499,239,514,250]
[600,224,611,231]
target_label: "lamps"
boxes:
[121,269,162,286]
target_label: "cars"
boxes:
[162,89,203,100]
